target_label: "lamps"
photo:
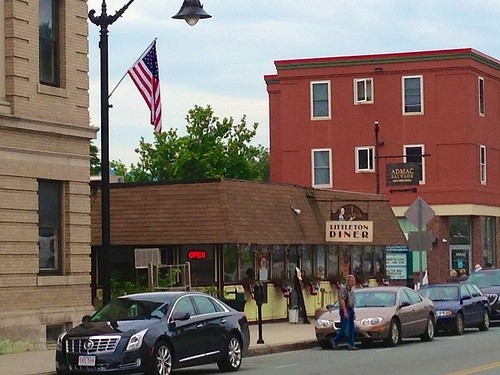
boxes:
[291,207,301,215]
[375,68,382,72]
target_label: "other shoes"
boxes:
[330,338,339,350]
[347,345,360,350]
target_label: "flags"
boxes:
[128,40,164,136]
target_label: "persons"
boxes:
[448,263,493,284]
[330,275,360,351]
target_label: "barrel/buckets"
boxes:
[288,306,299,323]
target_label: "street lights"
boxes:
[88,0,212,307]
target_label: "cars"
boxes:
[462,268,500,327]
[315,286,437,349]
[416,283,491,335]
[54,292,251,375]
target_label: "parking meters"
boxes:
[254,283,267,344]
